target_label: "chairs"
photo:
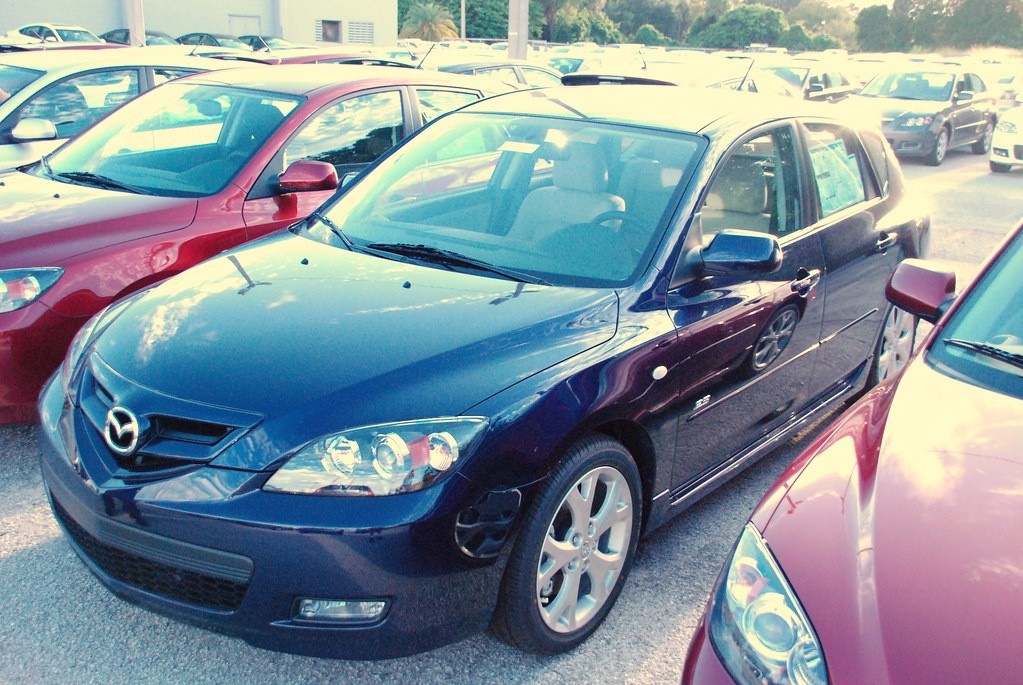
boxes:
[700,159,776,235]
[775,68,800,88]
[506,140,625,241]
[231,104,289,176]
[617,142,696,244]
[941,81,966,97]
[897,75,929,95]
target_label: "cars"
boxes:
[35,72,932,661]
[0,23,1019,192]
[0,58,487,433]
[677,205,1022,685]
